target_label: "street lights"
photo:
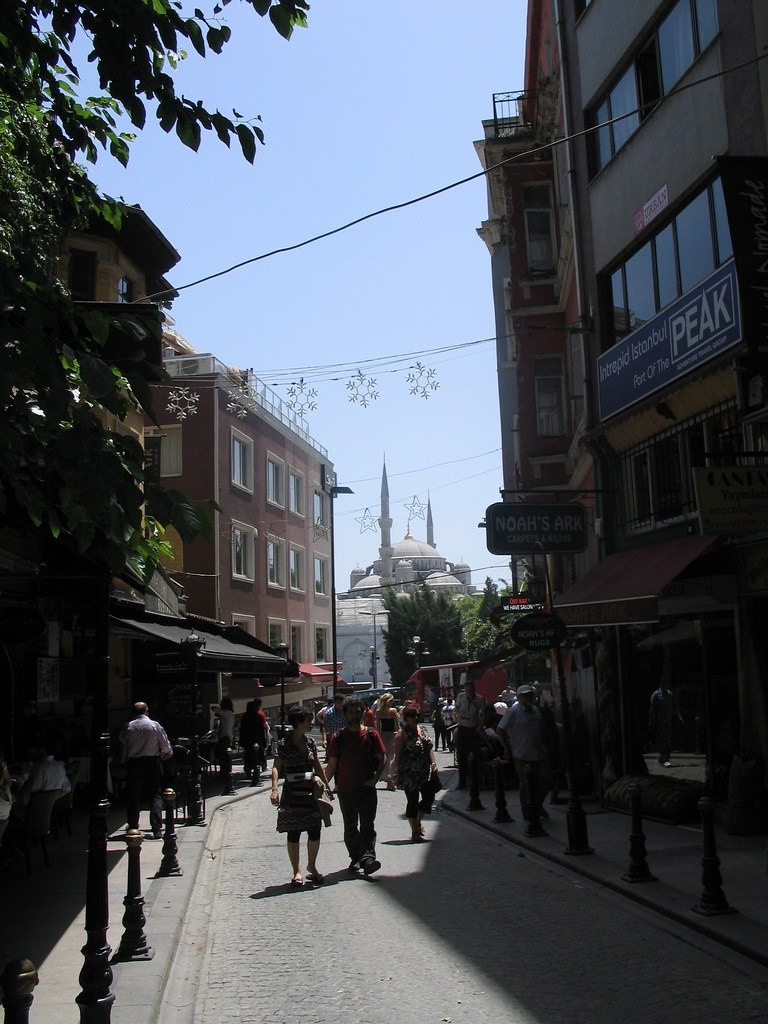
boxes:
[358,610,390,688]
[406,636,431,728]
[328,486,354,705]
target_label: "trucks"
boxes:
[402,659,482,725]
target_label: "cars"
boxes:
[355,686,408,717]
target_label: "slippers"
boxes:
[291,879,303,886]
[307,873,326,883]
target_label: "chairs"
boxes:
[0,732,240,876]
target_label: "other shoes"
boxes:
[523,805,551,820]
[411,825,426,842]
[153,831,162,838]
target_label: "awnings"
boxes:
[110,615,287,675]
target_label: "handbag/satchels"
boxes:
[430,763,444,795]
[289,782,315,810]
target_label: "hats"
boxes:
[517,684,535,696]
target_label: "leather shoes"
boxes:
[364,859,381,874]
[349,861,361,871]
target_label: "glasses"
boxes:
[408,714,421,718]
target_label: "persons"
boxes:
[323,693,347,742]
[647,679,684,768]
[388,706,437,841]
[4,743,72,869]
[0,761,14,821]
[271,706,333,885]
[254,698,266,725]
[211,698,235,795]
[432,696,446,751]
[239,701,264,786]
[120,700,174,838]
[451,681,486,789]
[323,699,388,875]
[373,692,399,790]
[317,699,335,724]
[497,685,549,819]
